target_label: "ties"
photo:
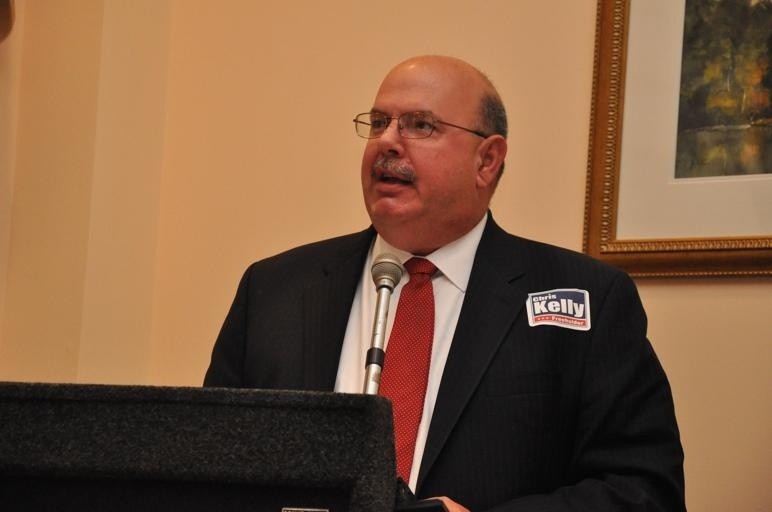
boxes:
[375,253,438,491]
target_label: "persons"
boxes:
[202,54,687,512]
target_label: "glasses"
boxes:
[350,109,492,143]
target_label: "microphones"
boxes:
[362,252,406,394]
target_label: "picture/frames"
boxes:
[582,0,772,278]
